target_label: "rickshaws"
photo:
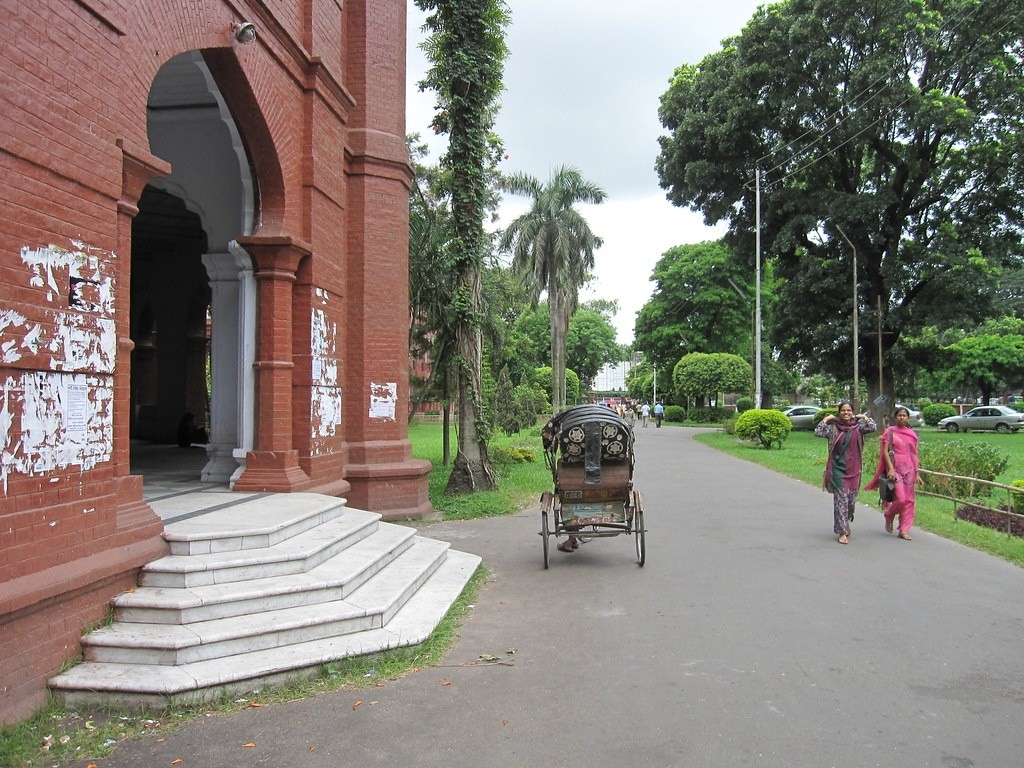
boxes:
[538,405,649,570]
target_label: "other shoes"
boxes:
[885,520,893,533]
[898,532,912,540]
[572,540,578,549]
[557,541,573,552]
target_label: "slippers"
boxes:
[838,536,848,544]
[846,528,851,536]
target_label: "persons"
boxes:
[616,402,636,429]
[557,535,579,552]
[636,402,642,420]
[815,401,877,543]
[654,401,663,428]
[641,402,650,428]
[865,407,923,540]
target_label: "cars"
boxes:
[937,406,1024,434]
[858,405,924,427]
[782,406,822,429]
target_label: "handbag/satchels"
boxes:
[879,473,897,502]
